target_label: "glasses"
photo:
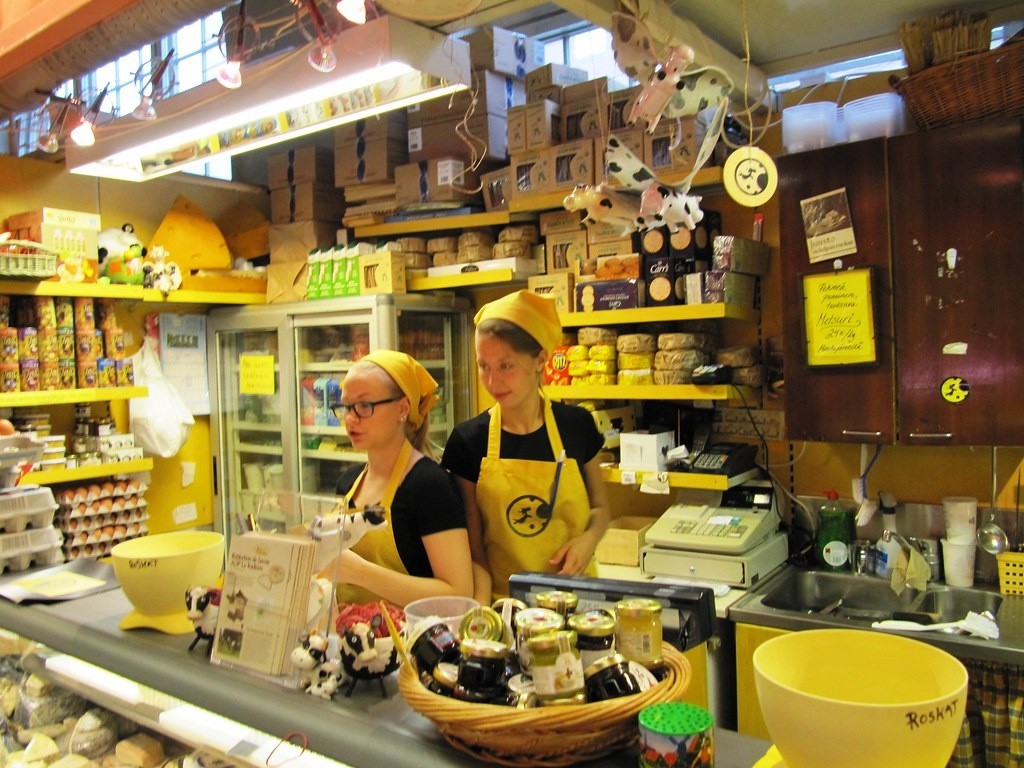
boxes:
[330,394,409,421]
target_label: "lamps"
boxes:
[131,48,174,121]
[336,0,366,24]
[70,83,109,146]
[37,93,72,153]
[215,0,247,89]
[305,0,337,72]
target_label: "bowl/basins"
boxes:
[110,530,226,615]
[751,628,969,767]
[781,93,906,155]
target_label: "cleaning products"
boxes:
[815,489,851,573]
[874,490,902,580]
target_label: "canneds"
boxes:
[65,401,117,468]
[352,328,445,362]
[0,293,134,393]
[406,590,662,705]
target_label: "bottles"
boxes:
[816,491,851,572]
[405,590,664,711]
[67,402,119,468]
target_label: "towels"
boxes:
[945,655,1024,768]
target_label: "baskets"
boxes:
[995,551,1024,597]
[378,601,692,768]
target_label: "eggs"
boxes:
[56,479,142,556]
[0,418,16,435]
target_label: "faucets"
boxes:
[851,539,871,577]
[881,529,934,587]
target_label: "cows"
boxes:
[338,613,405,697]
[291,629,345,700]
[185,585,219,651]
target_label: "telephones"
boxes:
[679,423,710,469]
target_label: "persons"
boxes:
[318,348,474,617]
[441,289,612,606]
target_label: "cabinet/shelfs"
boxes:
[508,167,760,490]
[350,210,535,292]
[0,281,258,562]
[774,108,1024,446]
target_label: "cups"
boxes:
[942,541,975,587]
[942,496,978,544]
[403,596,480,639]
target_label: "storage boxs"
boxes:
[270,66,712,312]
[595,516,657,566]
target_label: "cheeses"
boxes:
[0,673,172,768]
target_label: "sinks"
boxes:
[903,585,1003,638]
[833,571,925,625]
[729,565,855,630]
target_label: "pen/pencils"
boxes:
[544,449,565,522]
